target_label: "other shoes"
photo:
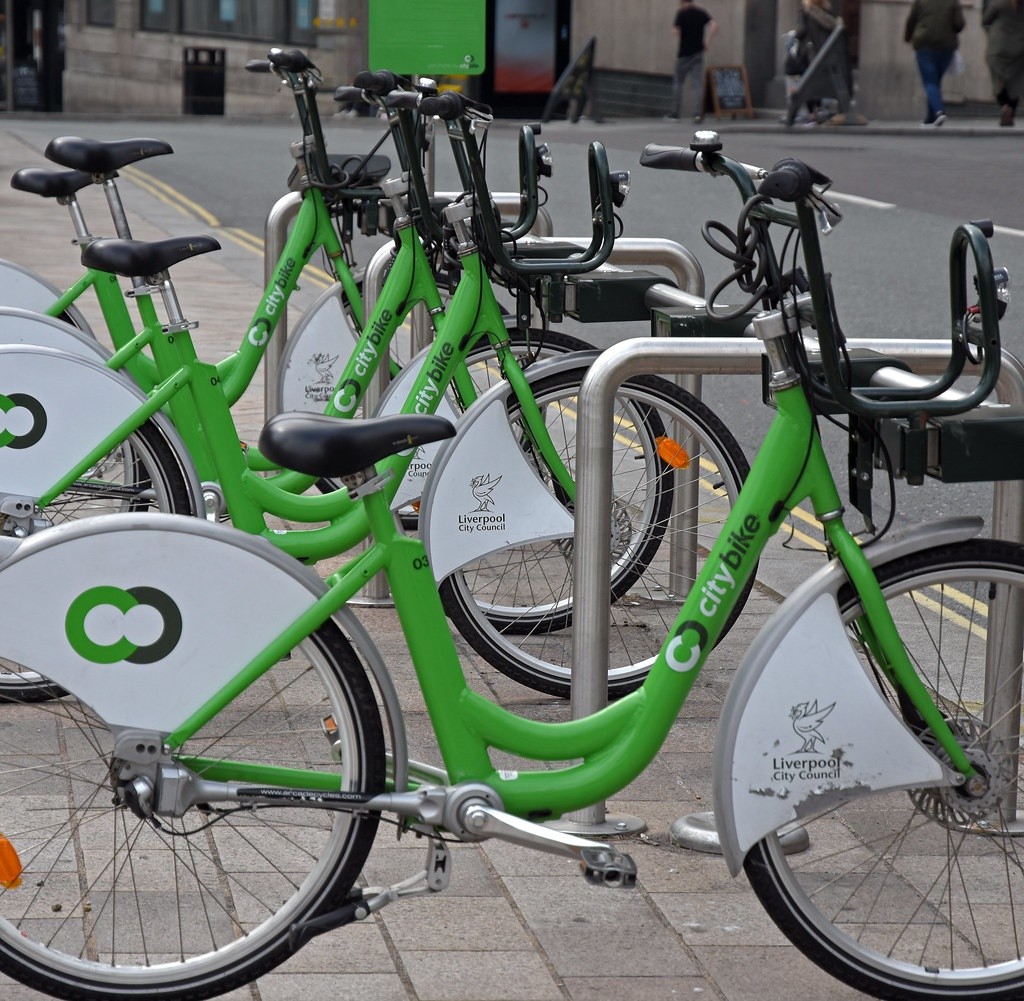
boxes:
[692,113,703,123]
[664,112,680,122]
[1000,107,1014,126]
[800,116,818,127]
[922,110,947,127]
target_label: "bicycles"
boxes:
[1,45,758,698]
[0,131,1024,1001]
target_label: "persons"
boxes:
[980,0,1024,127]
[779,1,856,126]
[903,0,965,126]
[664,0,716,123]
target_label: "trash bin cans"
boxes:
[180,45,228,115]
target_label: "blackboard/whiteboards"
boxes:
[705,63,754,114]
